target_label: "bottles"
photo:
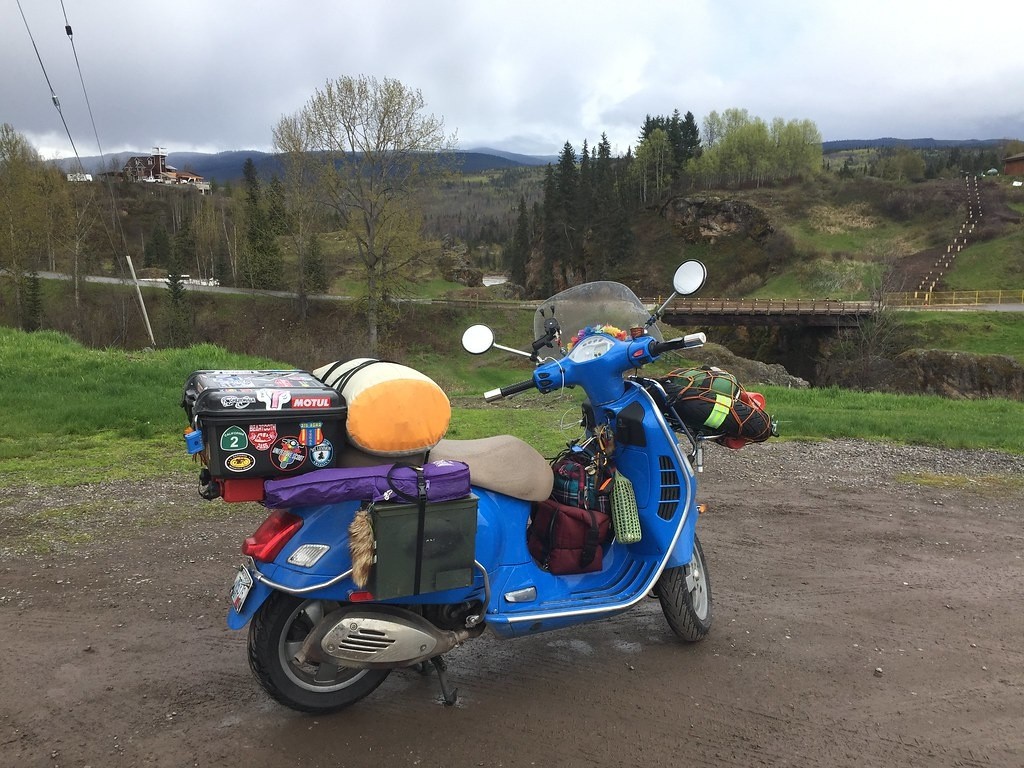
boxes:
[715,438,746,449]
[741,392,765,411]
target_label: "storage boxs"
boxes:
[361,490,479,601]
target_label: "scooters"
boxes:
[179,255,739,719]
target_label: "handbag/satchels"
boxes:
[528,499,611,575]
[549,424,610,514]
